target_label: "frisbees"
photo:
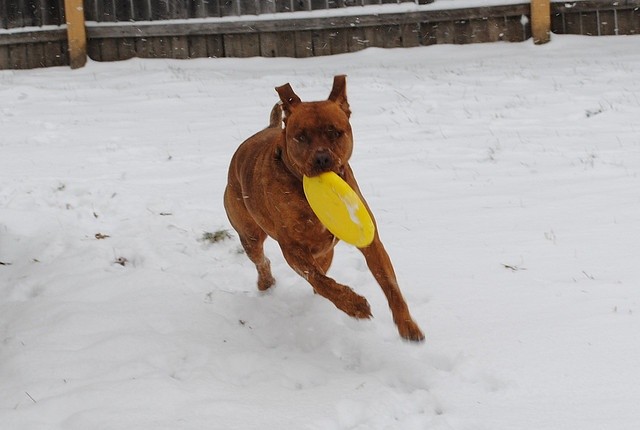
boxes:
[303,171,376,248]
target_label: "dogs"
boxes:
[222,71,427,345]
